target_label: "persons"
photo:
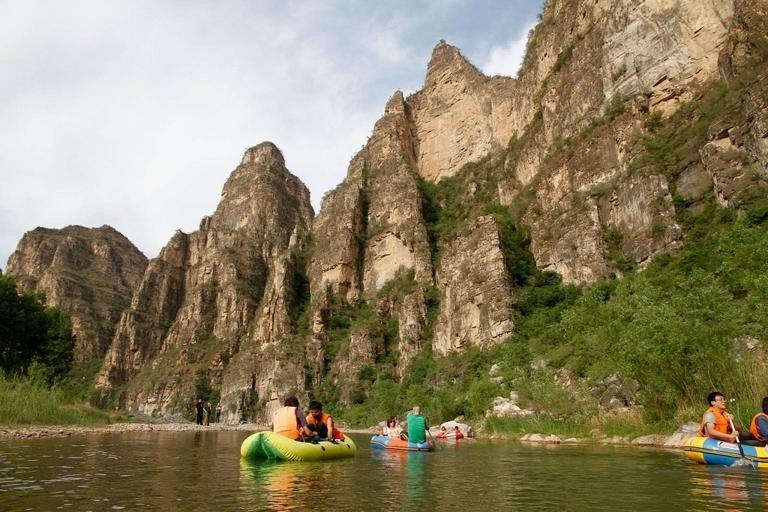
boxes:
[382,406,430,444]
[439,426,448,433]
[202,402,212,426]
[748,396,768,444]
[301,399,346,442]
[215,402,222,423]
[270,395,319,442]
[195,398,204,425]
[698,391,760,446]
[298,423,318,438]
[449,425,460,433]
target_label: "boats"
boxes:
[684,437,768,468]
[370,436,436,451]
[241,431,357,462]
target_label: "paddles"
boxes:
[728,413,748,458]
